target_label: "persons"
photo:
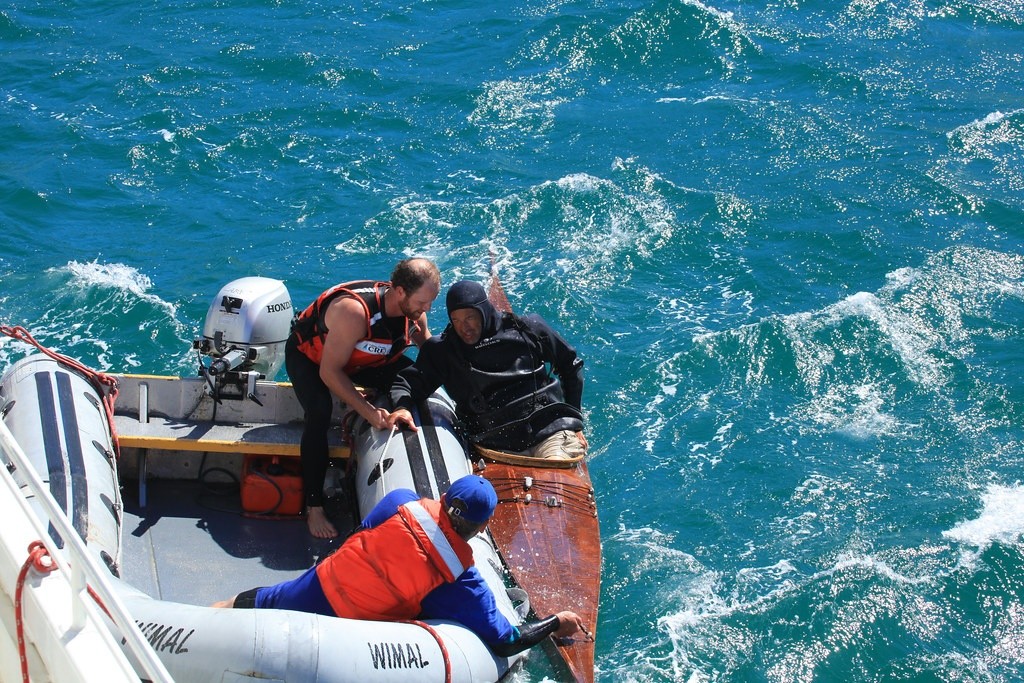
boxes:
[206,475,585,659]
[387,280,584,452]
[284,256,440,539]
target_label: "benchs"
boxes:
[109,411,354,509]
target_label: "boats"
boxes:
[471,255,601,683]
[0,277,530,683]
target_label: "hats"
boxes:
[444,474,497,521]
[446,279,500,335]
